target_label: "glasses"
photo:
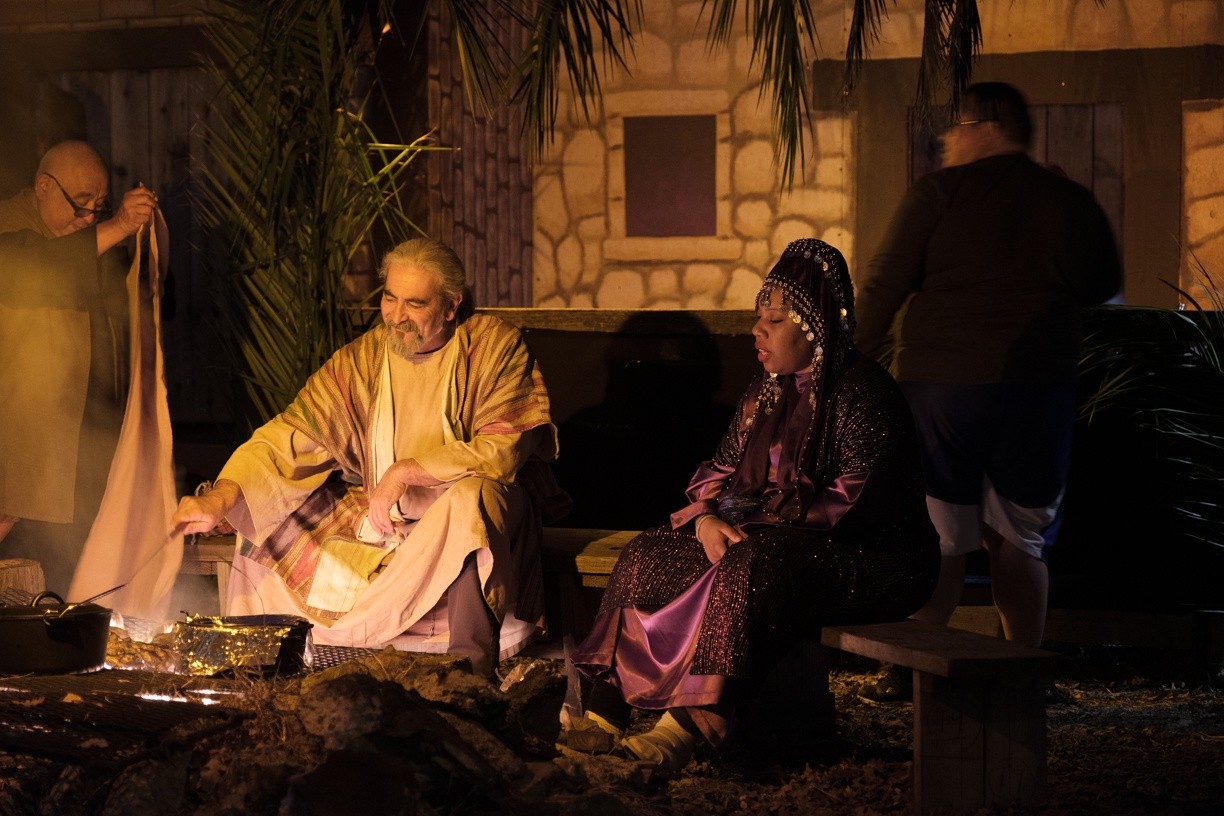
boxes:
[947,116,991,129]
[44,172,113,219]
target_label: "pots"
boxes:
[176,612,315,681]
[1,590,113,674]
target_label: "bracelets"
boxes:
[694,513,718,543]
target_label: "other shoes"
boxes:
[621,709,695,775]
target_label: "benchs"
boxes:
[183,515,1057,812]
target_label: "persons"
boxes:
[552,235,907,773]
[173,234,562,696]
[849,82,1129,654]
[1,135,176,616]
